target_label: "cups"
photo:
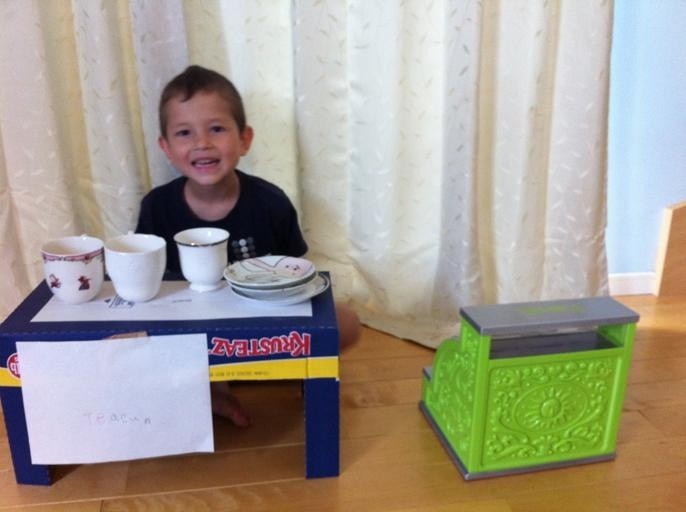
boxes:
[40,232,166,302]
[173,227,232,292]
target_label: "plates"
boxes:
[223,254,330,305]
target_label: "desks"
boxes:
[1,271,341,486]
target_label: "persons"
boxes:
[134,65,361,425]
